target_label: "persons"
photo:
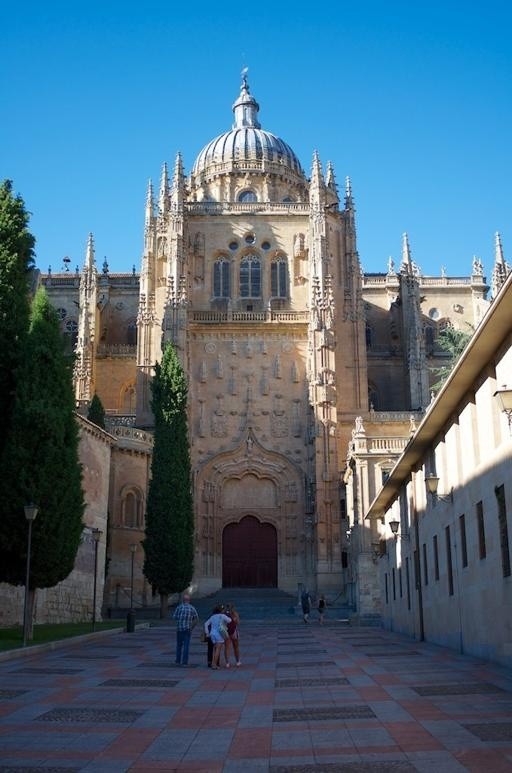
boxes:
[172,593,200,666]
[315,591,328,626]
[223,602,242,669]
[204,605,232,669]
[299,586,313,625]
[203,603,225,669]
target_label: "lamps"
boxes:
[424,472,454,504]
[370,540,390,560]
[493,384,512,438]
[389,518,410,542]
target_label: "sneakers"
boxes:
[176,662,192,667]
[224,662,230,668]
[208,665,222,672]
[303,618,324,626]
[236,661,242,666]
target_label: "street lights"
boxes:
[92,526,106,634]
[18,503,44,642]
[127,541,142,609]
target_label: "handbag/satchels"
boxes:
[232,630,240,640]
[199,631,209,642]
[218,624,229,640]
[318,607,329,613]
